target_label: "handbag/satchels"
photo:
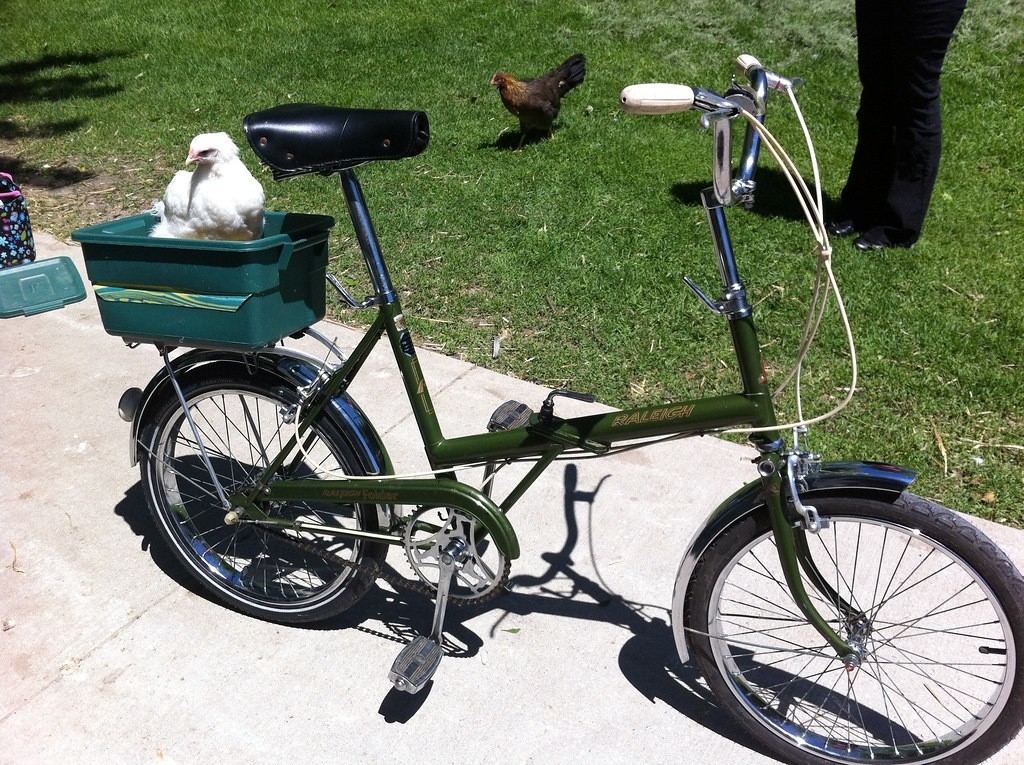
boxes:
[0,172,36,269]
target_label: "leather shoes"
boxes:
[827,219,860,237]
[852,227,891,250]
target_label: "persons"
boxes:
[825,0,969,251]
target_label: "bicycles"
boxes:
[72,51,1024,765]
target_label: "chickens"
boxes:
[147,130,266,241]
[489,52,586,140]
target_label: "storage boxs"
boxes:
[71,209,336,349]
